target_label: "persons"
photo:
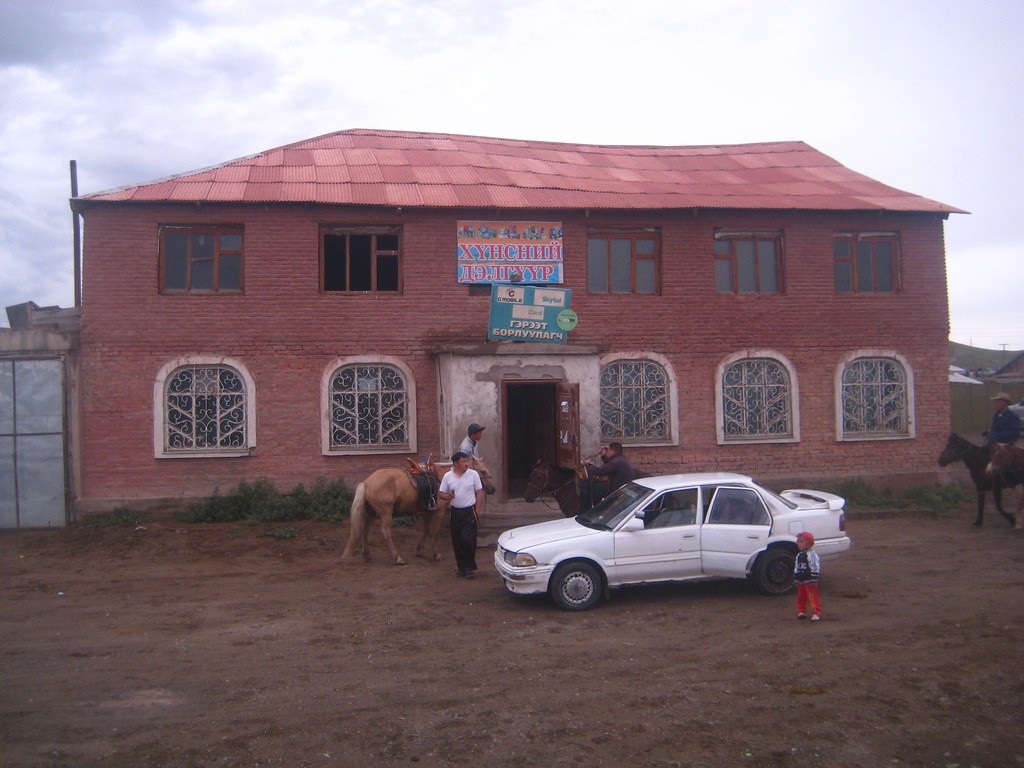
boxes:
[985,391,1020,473]
[792,531,822,621]
[583,440,637,495]
[456,421,485,471]
[438,452,483,579]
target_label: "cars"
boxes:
[494,472,849,610]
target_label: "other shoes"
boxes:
[810,614,820,621]
[797,612,807,618]
[466,568,479,577]
[455,572,465,577]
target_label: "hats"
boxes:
[799,532,814,546]
[468,424,485,434]
[990,391,1013,405]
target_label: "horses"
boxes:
[524,460,653,518]
[341,455,495,564]
[937,430,1024,528]
